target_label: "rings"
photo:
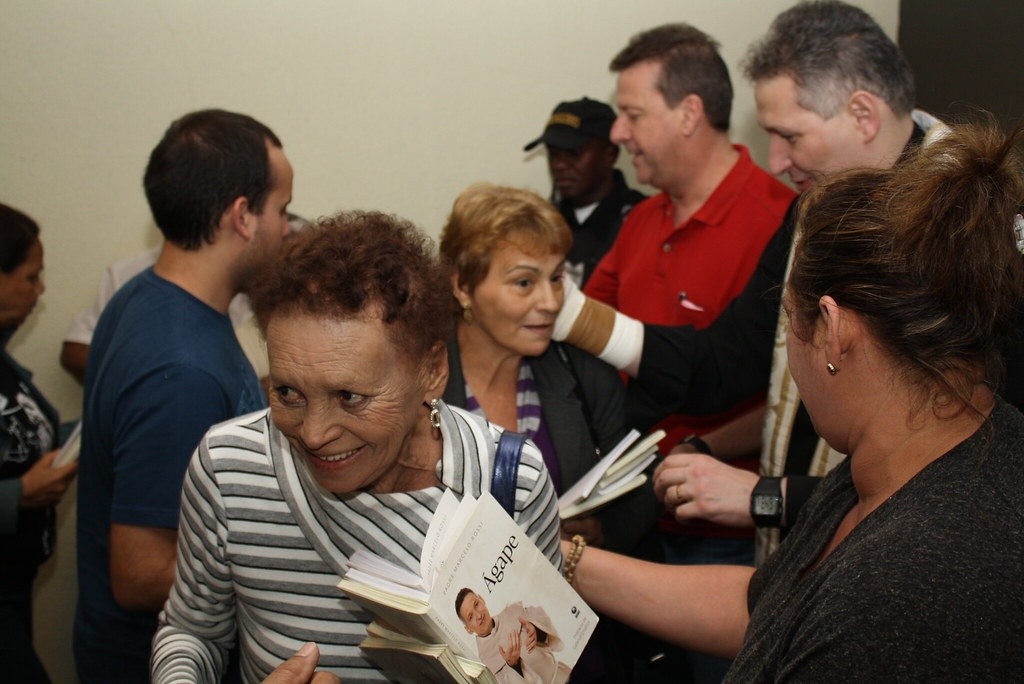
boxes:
[676,485,684,499]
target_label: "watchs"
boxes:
[751,476,786,529]
[680,434,711,455]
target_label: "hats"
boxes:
[524,97,618,150]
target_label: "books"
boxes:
[53,420,81,469]
[337,487,599,684]
[559,429,666,518]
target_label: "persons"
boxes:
[560,128,1024,684]
[60,243,271,396]
[0,202,77,683]
[522,98,648,279]
[585,21,797,684]
[148,213,566,684]
[73,108,293,683]
[549,0,951,567]
[432,185,659,684]
[456,589,571,684]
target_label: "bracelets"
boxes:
[562,537,577,577]
[567,536,584,584]
